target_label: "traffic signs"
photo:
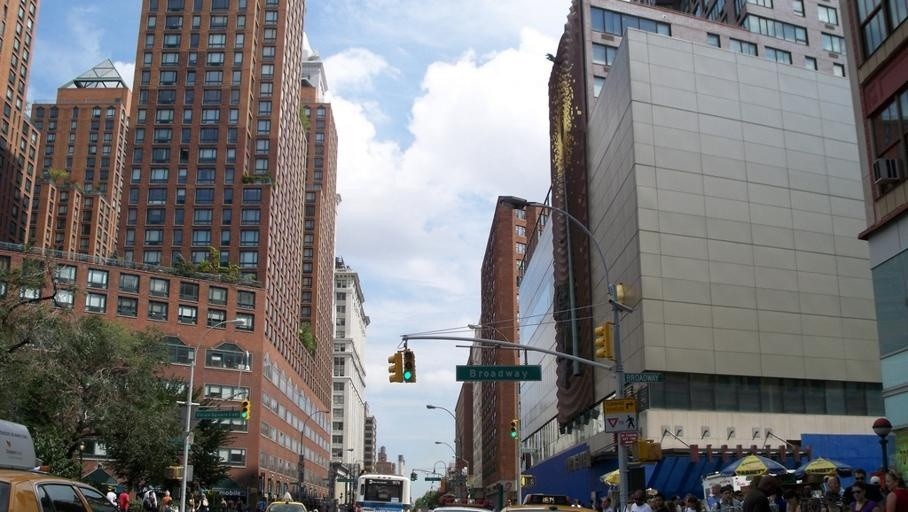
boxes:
[337,479,355,483]
[194,410,240,418]
[455,364,542,382]
[425,478,442,481]
[624,372,669,384]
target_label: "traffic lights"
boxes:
[166,465,184,480]
[511,419,520,439]
[387,351,403,384]
[403,350,417,384]
[240,400,251,419]
[520,474,537,488]
[629,438,649,461]
[411,472,416,481]
[594,322,612,361]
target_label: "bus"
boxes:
[353,471,412,512]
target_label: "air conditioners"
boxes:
[872,158,900,184]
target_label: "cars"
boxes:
[426,504,493,512]
[0,418,124,512]
[498,492,600,512]
[264,491,308,512]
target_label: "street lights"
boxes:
[434,441,457,456]
[467,322,524,506]
[431,460,448,494]
[178,318,246,512]
[298,410,331,500]
[78,439,85,482]
[494,194,729,512]
[870,415,894,474]
[329,448,356,498]
[425,404,463,498]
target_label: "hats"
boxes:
[869,476,881,485]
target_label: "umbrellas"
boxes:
[793,458,850,490]
[719,453,787,487]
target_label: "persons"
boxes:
[104,481,356,512]
[504,463,908,511]
